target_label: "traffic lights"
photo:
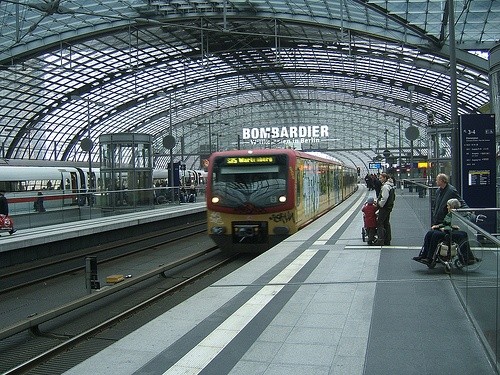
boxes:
[357,166,361,176]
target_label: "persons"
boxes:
[413,198,467,264]
[0,193,16,237]
[361,172,395,245]
[33,177,197,213]
[451,229,482,264]
[432,174,473,228]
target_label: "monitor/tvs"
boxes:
[369,163,381,169]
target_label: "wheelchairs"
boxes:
[424,213,487,274]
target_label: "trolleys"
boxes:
[361,201,380,241]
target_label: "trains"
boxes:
[205,147,358,249]
[0,164,208,215]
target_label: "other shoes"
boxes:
[475,257,481,261]
[372,240,385,245]
[468,259,477,264]
[384,240,390,245]
[413,256,422,261]
[421,258,430,264]
